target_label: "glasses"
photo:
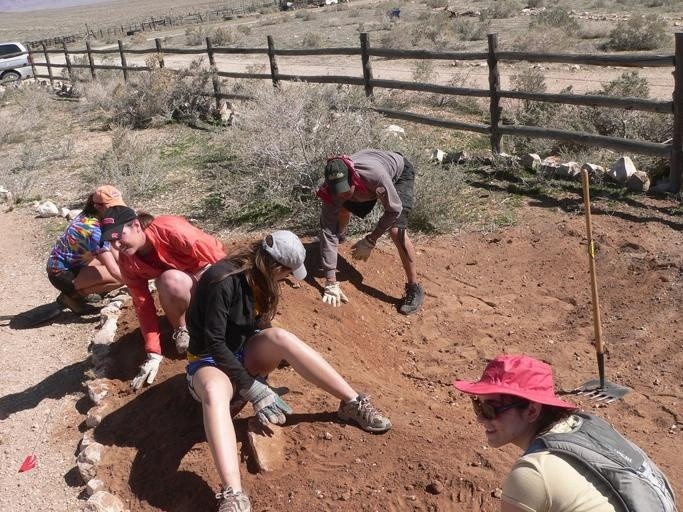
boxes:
[470,397,526,419]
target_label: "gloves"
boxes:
[239,379,292,425]
[322,279,348,308]
[129,352,163,389]
[351,235,375,262]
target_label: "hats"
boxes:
[262,229,307,281]
[98,205,136,248]
[325,159,351,196]
[92,185,127,208]
[451,355,578,411]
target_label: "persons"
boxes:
[186,231,392,512]
[453,353,675,512]
[319,147,424,313]
[48,184,126,318]
[99,208,228,389]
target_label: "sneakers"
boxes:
[399,283,423,314]
[171,326,189,353]
[215,484,250,512]
[337,394,392,432]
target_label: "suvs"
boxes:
[0,41,33,91]
